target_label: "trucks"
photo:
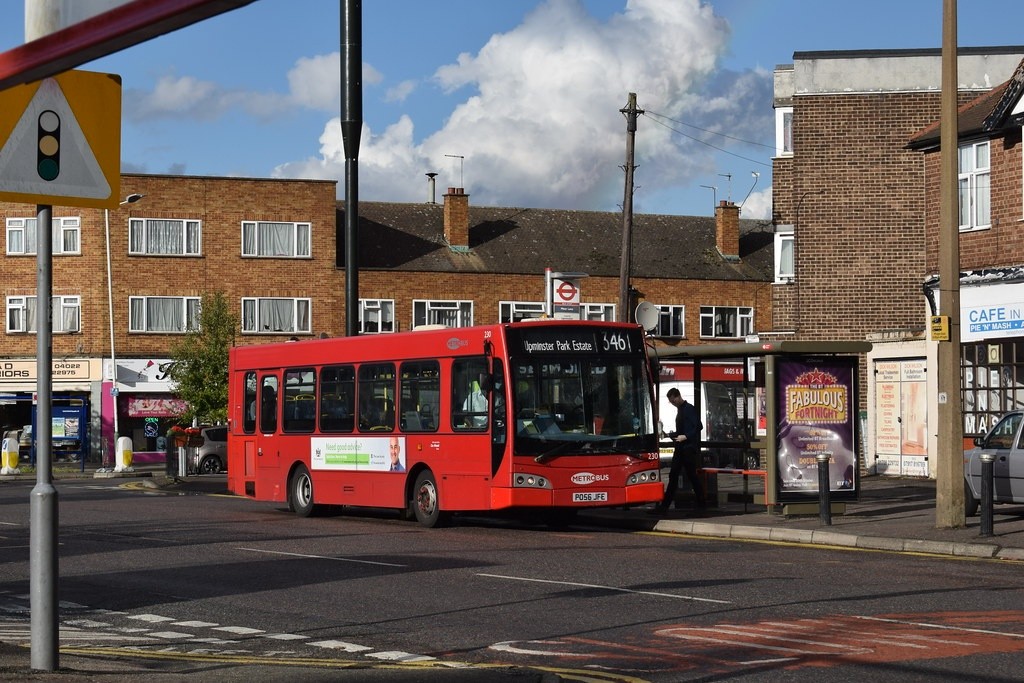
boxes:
[647,382,737,468]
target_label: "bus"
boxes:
[227,320,664,529]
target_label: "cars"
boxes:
[964,410,1024,516]
[708,419,760,470]
[189,425,227,474]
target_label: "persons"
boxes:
[653,388,708,514]
[462,371,506,437]
[249,384,276,424]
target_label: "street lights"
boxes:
[105,193,142,444]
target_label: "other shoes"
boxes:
[652,505,669,512]
[697,502,705,514]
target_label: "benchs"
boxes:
[695,468,768,505]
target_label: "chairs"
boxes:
[268,395,585,433]
